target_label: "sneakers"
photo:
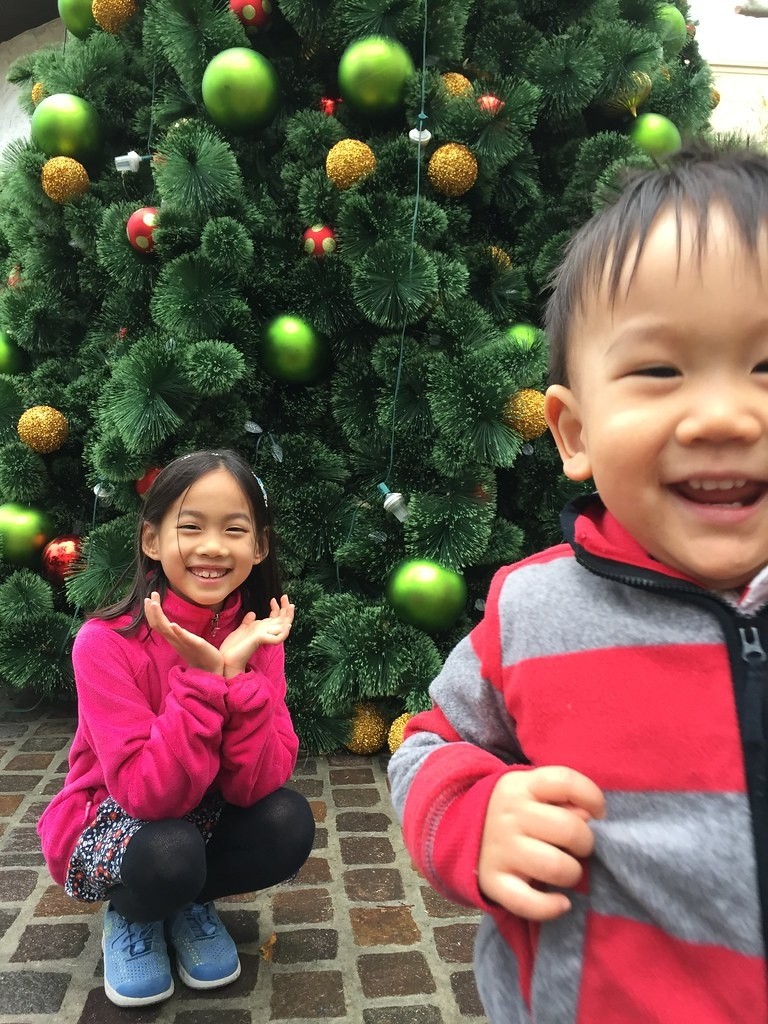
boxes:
[101,898,174,1007]
[165,900,241,989]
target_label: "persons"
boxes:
[388,140,768,1024]
[35,453,315,1007]
